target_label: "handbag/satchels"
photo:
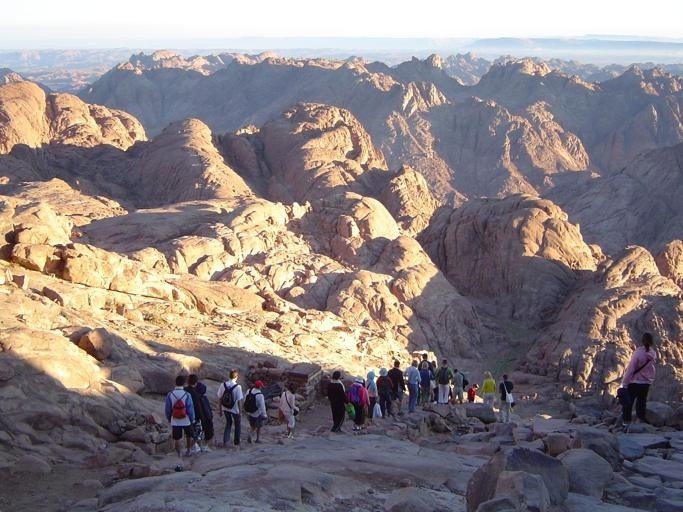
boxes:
[293,408,299,416]
[506,393,513,402]
[463,380,468,387]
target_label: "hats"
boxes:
[256,380,264,388]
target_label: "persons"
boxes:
[165,375,195,472]
[325,353,515,433]
[195,382,214,452]
[277,382,300,438]
[243,380,268,443]
[216,369,244,448]
[183,373,207,455]
[616,332,657,434]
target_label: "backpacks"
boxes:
[222,382,238,409]
[439,366,449,385]
[171,391,187,418]
[244,388,262,412]
